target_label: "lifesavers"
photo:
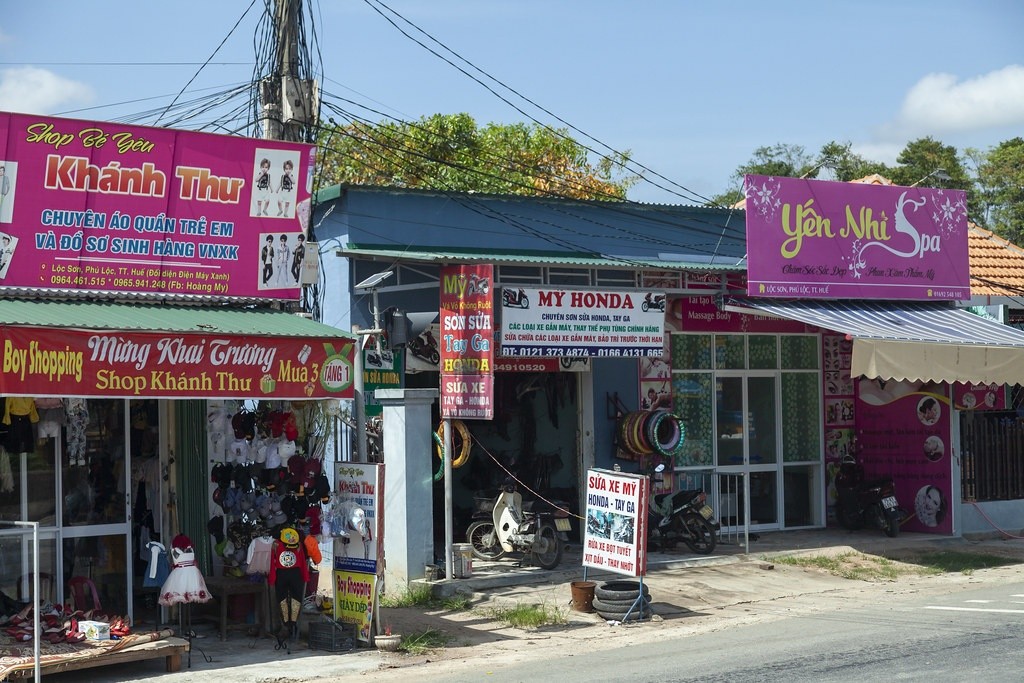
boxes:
[432,430,445,482]
[437,419,471,468]
[615,409,685,457]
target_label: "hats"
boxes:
[208,408,330,564]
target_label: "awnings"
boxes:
[0,299,353,339]
[337,247,748,269]
[732,300,1024,347]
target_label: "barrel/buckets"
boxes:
[452,543,474,578]
[570,582,597,613]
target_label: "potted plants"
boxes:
[375,634,401,652]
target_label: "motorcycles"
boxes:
[366,352,383,369]
[493,451,572,546]
[642,290,666,313]
[588,512,613,540]
[381,351,393,362]
[632,463,719,554]
[502,287,530,309]
[464,273,491,298]
[560,357,589,368]
[837,460,900,537]
[408,325,440,366]
[614,517,634,544]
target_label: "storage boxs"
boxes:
[308,619,358,653]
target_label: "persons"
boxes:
[269,534,308,642]
[911,388,997,533]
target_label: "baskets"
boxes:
[473,487,497,512]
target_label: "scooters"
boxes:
[465,447,559,569]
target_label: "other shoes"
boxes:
[5,598,130,646]
